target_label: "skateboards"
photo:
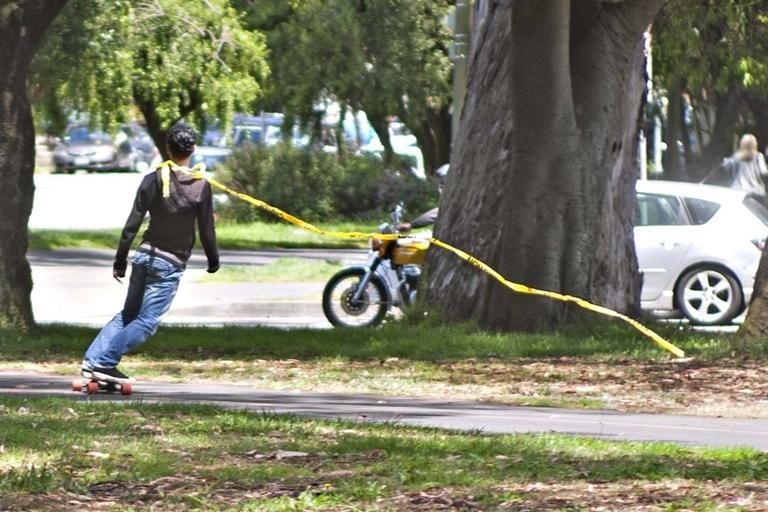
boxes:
[72,371,139,395]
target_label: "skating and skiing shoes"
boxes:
[72,368,132,395]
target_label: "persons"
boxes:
[398,163,453,233]
[721,132,768,196]
[78,125,222,383]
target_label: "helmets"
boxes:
[436,163,450,176]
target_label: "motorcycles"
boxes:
[323,202,432,329]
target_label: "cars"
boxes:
[635,179,767,325]
[54,126,156,173]
[189,101,422,171]
